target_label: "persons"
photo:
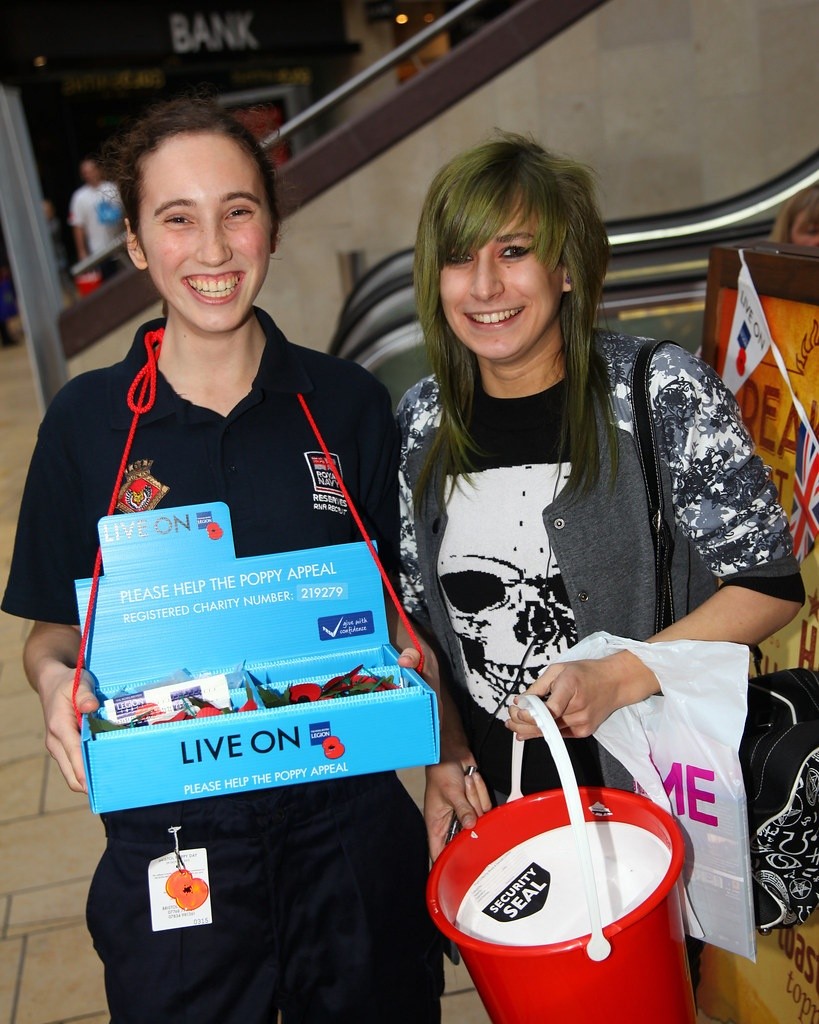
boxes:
[67,157,128,282]
[0,93,463,1024]
[389,145,804,1017]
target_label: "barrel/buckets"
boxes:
[424,693,700,1024]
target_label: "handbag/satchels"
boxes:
[538,631,757,964]
[737,667,819,936]
